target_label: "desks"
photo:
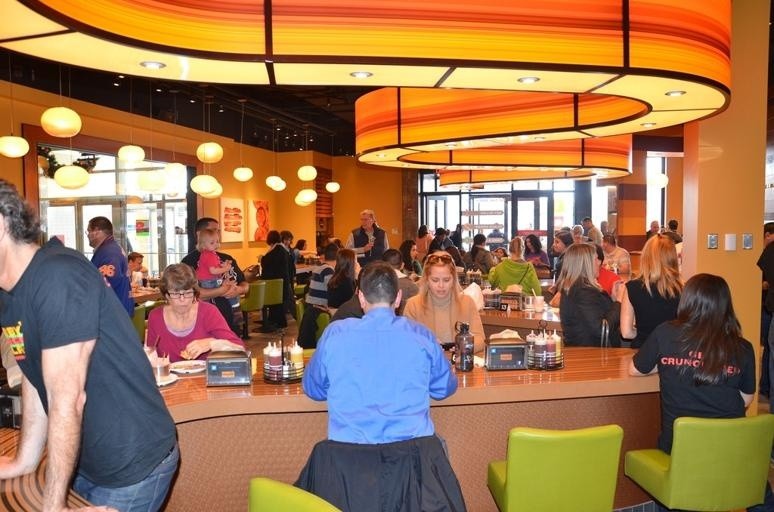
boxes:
[459,278,562,348]
[0,345,662,511]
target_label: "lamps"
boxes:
[41,107,82,138]
[197,141,224,164]
[297,165,317,181]
[640,122,656,127]
[299,188,317,202]
[326,182,340,193]
[200,180,223,199]
[0,135,29,158]
[140,61,165,69]
[190,174,217,194]
[265,175,280,187]
[118,144,145,164]
[295,195,311,206]
[517,76,540,82]
[233,167,253,181]
[664,90,686,97]
[273,180,286,191]
[54,165,89,189]
[350,71,373,78]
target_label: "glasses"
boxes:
[166,290,195,299]
[425,254,455,267]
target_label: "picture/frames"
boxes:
[219,197,244,243]
[247,199,270,242]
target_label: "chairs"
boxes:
[624,411,774,511]
[295,297,305,327]
[130,305,145,344]
[264,279,283,306]
[240,280,267,312]
[314,312,330,341]
[486,425,625,511]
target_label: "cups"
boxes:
[534,296,544,313]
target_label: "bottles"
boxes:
[291,341,303,378]
[546,331,556,369]
[264,342,273,381]
[526,329,537,369]
[535,332,546,368]
[455,323,474,372]
[269,343,282,381]
[552,329,561,364]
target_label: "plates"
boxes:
[171,360,207,373]
[154,374,178,387]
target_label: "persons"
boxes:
[580,217,602,244]
[446,245,482,272]
[464,234,492,271]
[603,236,630,274]
[416,225,434,254]
[403,250,485,355]
[764,222,774,246]
[1,177,180,511]
[450,224,464,250]
[572,225,594,245]
[551,242,632,347]
[195,229,242,315]
[383,248,407,278]
[280,231,297,321]
[400,239,422,274]
[523,235,551,274]
[554,231,573,282]
[443,229,454,248]
[293,238,317,264]
[630,273,755,455]
[127,252,146,279]
[756,240,774,404]
[298,242,339,308]
[489,237,541,295]
[646,221,661,241]
[494,247,508,262]
[146,264,246,361]
[345,209,389,265]
[429,228,446,252]
[181,218,249,338]
[664,218,683,243]
[257,230,296,338]
[327,250,362,317]
[601,221,616,244]
[330,269,364,322]
[487,223,508,249]
[592,244,624,301]
[88,216,136,318]
[621,234,684,348]
[302,261,458,444]
[318,234,343,256]
